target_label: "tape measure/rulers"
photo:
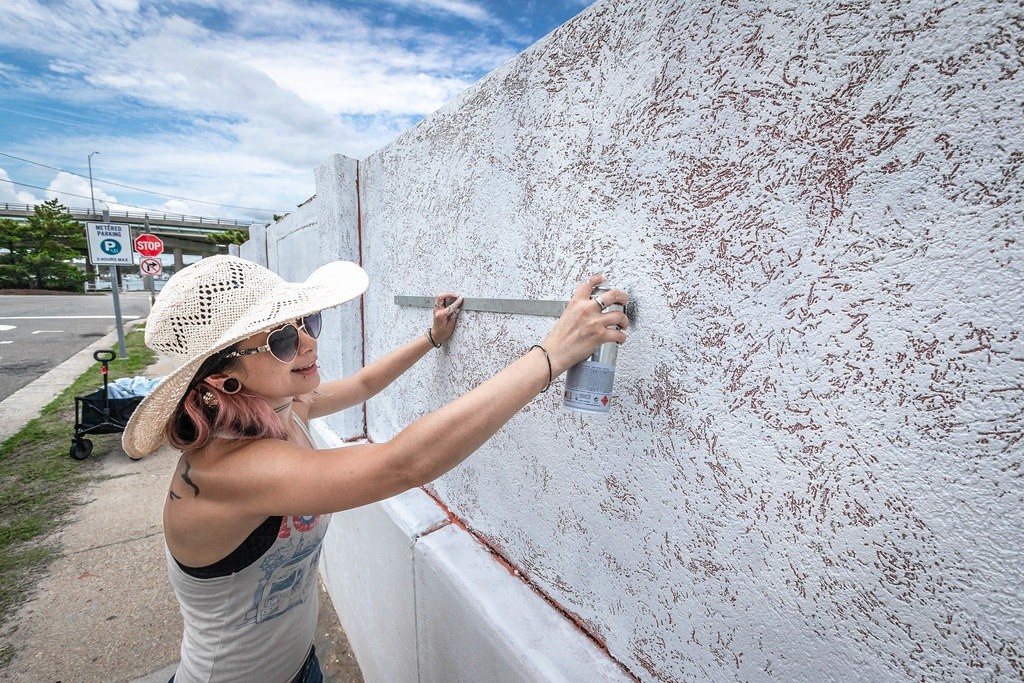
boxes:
[392,293,571,318]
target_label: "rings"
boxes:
[595,297,606,310]
[585,277,598,290]
[434,301,443,307]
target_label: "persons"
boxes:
[145,255,629,683]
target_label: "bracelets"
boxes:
[426,328,442,348]
[530,345,552,393]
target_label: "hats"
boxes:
[122,254,370,459]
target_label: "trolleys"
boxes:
[67,350,174,461]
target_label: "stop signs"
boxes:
[134,232,164,258]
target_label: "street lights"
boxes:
[88,150,100,216]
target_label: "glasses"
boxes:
[223,310,322,364]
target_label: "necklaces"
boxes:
[273,400,293,414]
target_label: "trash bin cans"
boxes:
[143,274,151,289]
